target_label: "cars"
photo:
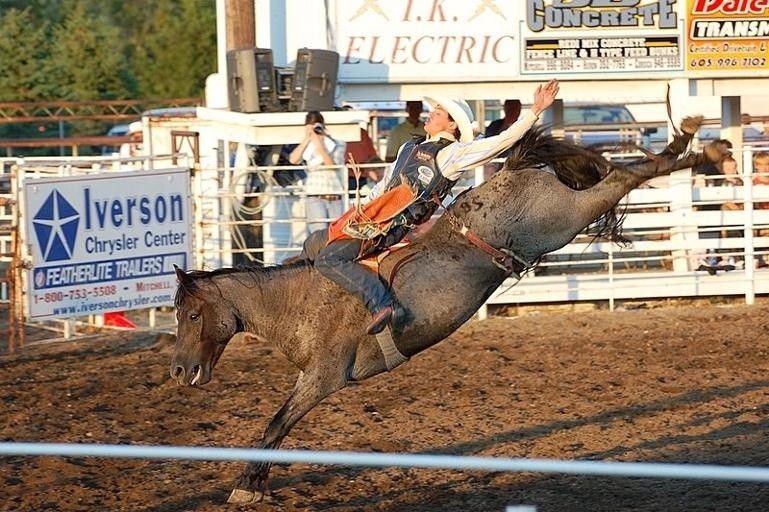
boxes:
[102,124,129,156]
[543,106,658,148]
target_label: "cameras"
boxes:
[313,122,323,134]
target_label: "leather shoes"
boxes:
[363,300,403,334]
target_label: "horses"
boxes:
[171,115,733,507]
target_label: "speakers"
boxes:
[288,47,340,112]
[226,47,277,112]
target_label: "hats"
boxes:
[423,94,475,145]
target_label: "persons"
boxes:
[696,114,769,269]
[288,111,345,234]
[386,101,427,162]
[119,121,143,164]
[359,156,385,196]
[301,79,560,334]
[345,105,376,198]
[485,101,522,172]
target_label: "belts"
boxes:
[307,194,341,200]
